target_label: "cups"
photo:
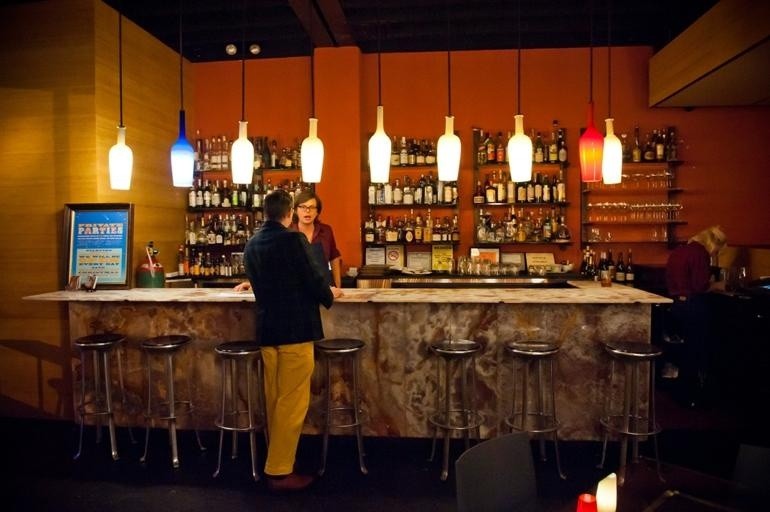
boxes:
[600,271,612,287]
[651,225,671,242]
[437,255,520,276]
[587,226,613,242]
[528,264,562,277]
[85,274,98,292]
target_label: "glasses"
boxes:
[295,203,318,212]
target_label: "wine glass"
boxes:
[588,200,681,222]
[589,170,676,191]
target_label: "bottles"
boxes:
[599,248,636,283]
[581,245,596,278]
[365,206,461,243]
[735,267,750,291]
[391,135,437,166]
[193,129,301,172]
[476,128,514,165]
[176,176,312,276]
[529,119,566,163]
[478,205,570,243]
[369,172,457,206]
[473,166,568,204]
[622,125,678,163]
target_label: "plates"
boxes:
[404,271,432,276]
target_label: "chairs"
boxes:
[455,432,538,511]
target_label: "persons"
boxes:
[664,225,727,300]
[233,188,342,292]
[241,191,343,490]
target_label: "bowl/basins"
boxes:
[563,263,575,273]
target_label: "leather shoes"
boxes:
[269,472,313,492]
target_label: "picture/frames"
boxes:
[67,202,135,290]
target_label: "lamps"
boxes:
[576,493,597,512]
[596,472,619,512]
